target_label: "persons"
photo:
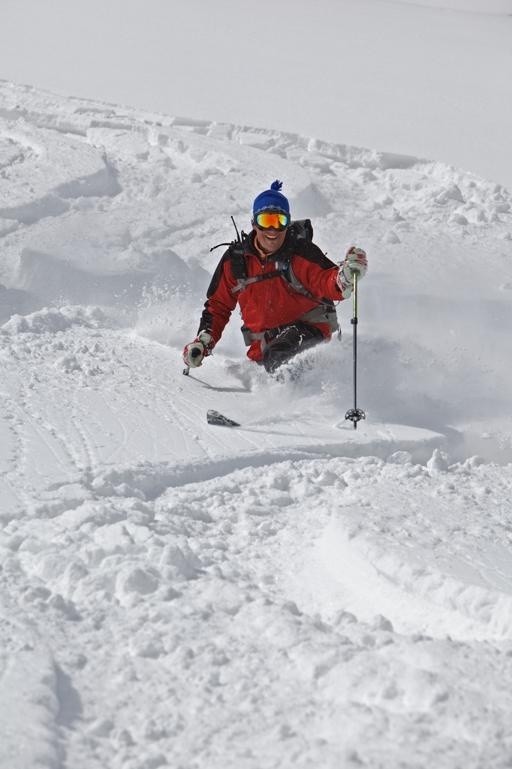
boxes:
[184,178,368,383]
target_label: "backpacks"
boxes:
[210,218,342,352]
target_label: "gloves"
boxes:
[183,329,216,368]
[336,246,368,299]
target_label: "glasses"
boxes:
[253,212,290,230]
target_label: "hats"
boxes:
[253,180,289,216]
